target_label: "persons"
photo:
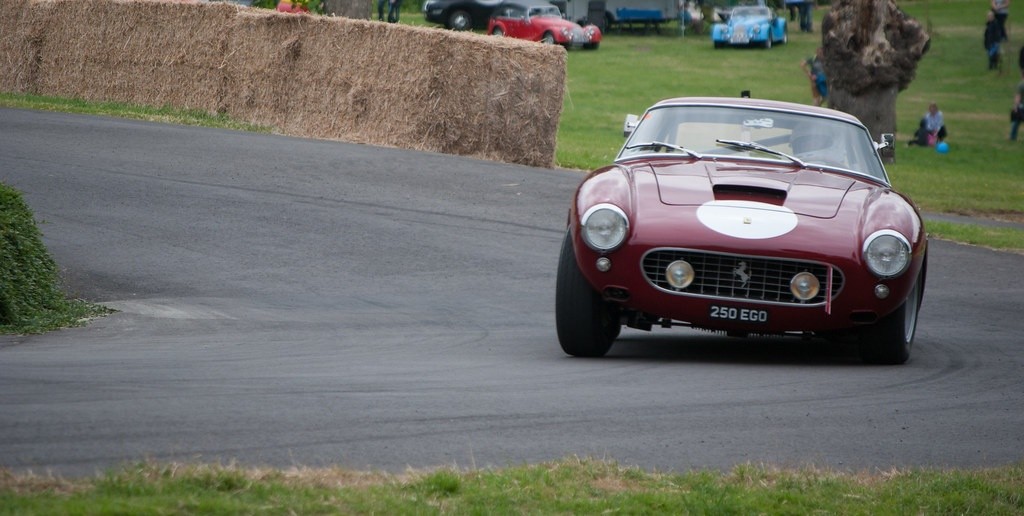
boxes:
[908,119,934,147]
[926,103,947,143]
[685,0,705,34]
[388,0,400,23]
[378,0,386,21]
[788,122,847,167]
[1010,76,1024,142]
[984,0,1010,71]
[783,0,818,34]
[802,48,832,107]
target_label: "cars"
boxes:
[711,4,789,50]
[420,0,514,31]
[487,0,603,52]
[552,88,930,366]
